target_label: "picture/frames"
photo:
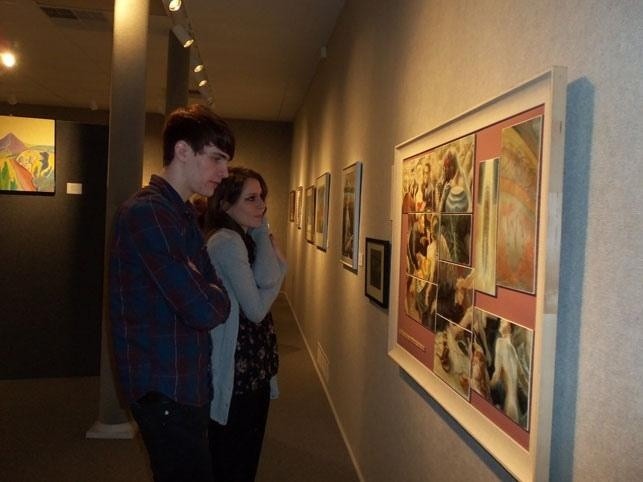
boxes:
[296,186,302,229]
[302,184,314,243]
[313,172,329,250]
[339,160,361,271]
[290,191,295,222]
[387,64,566,482]
[364,237,390,309]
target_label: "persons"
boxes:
[402,147,522,416]
[193,166,290,481]
[105,102,236,479]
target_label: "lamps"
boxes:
[162,0,216,106]
